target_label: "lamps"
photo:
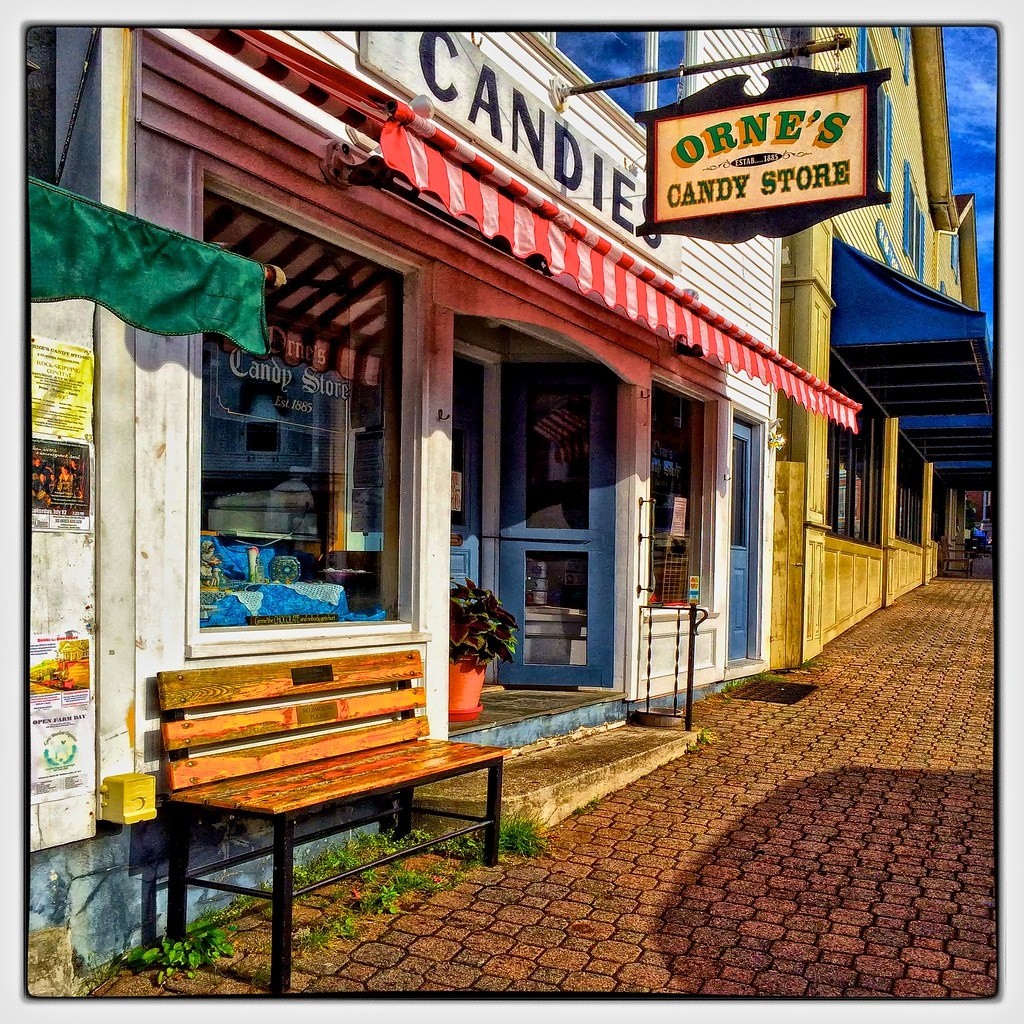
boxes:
[769,416,788,453]
[409,95,435,119]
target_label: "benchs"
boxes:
[156,649,512,989]
[940,536,975,579]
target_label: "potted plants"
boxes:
[448,576,519,723]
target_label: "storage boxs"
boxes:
[545,560,588,604]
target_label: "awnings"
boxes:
[27,176,288,356]
[831,236,993,493]
[323,94,863,435]
